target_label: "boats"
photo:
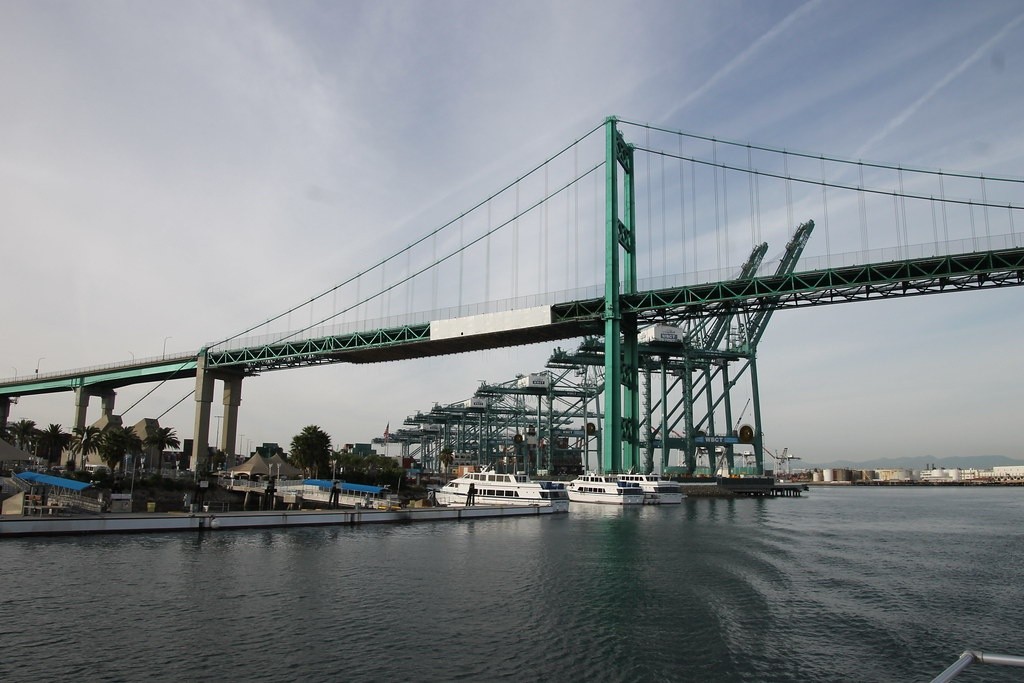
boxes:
[565,464,645,504]
[300,479,402,509]
[433,461,571,513]
[614,472,682,504]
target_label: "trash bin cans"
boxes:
[147,502,155,512]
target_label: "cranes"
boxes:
[370,215,816,474]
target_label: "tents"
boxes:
[227,452,303,479]
